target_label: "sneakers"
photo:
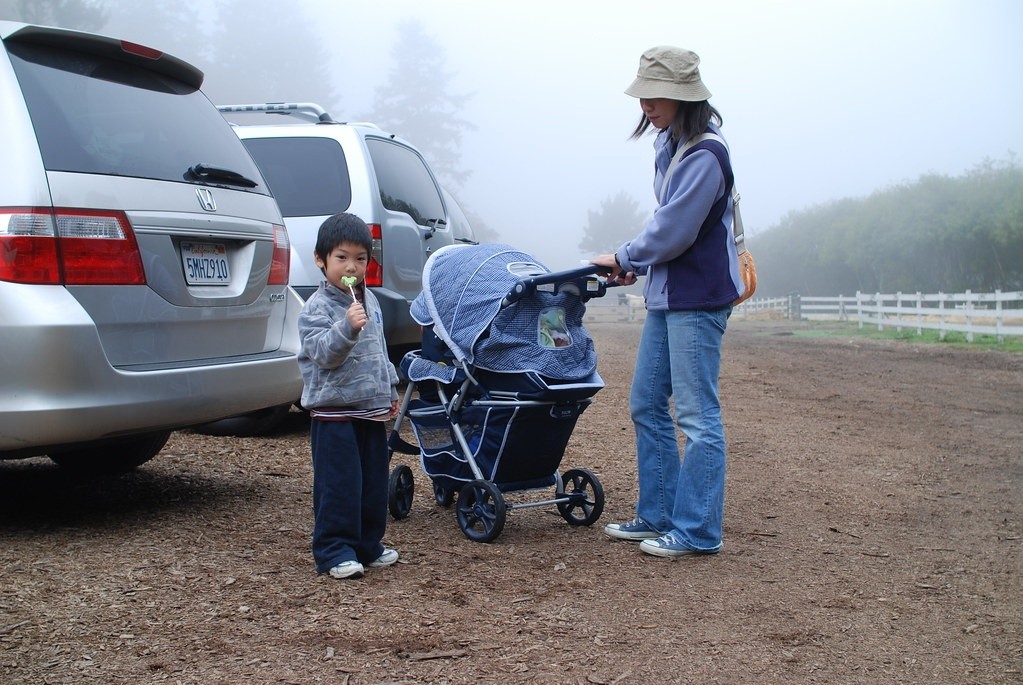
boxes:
[329,560,364,579]
[640,533,719,558]
[604,516,666,540]
[366,548,398,568]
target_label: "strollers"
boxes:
[386,242,637,544]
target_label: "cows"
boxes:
[618,291,645,319]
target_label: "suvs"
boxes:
[187,100,476,436]
[1,18,309,479]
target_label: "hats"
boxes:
[624,46,711,102]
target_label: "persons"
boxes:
[297,212,402,579]
[589,45,745,559]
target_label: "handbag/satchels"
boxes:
[730,247,757,306]
